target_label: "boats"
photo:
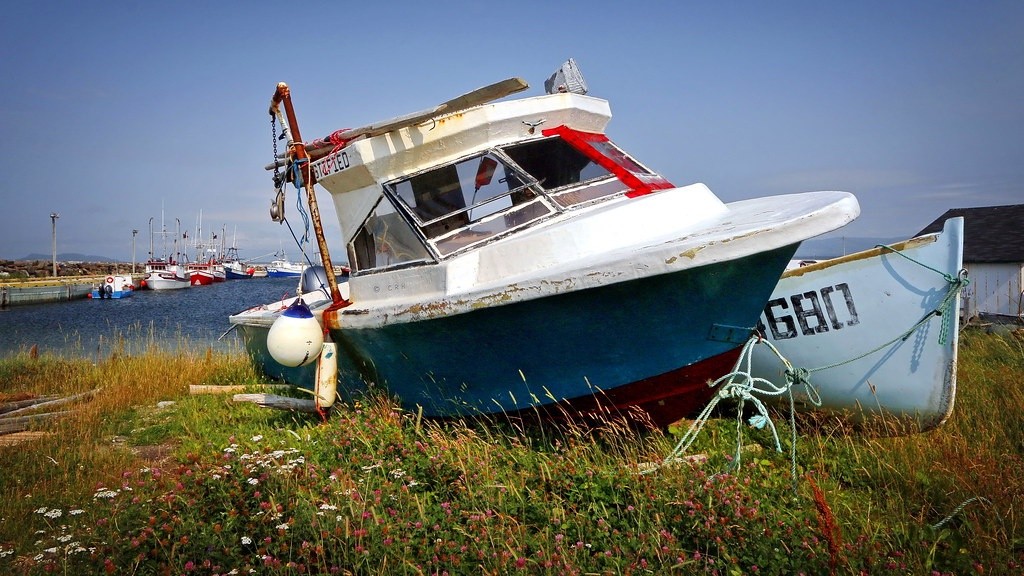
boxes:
[266,249,308,279]
[331,265,343,276]
[706,215,965,440]
[221,223,255,280]
[246,264,268,279]
[143,263,193,291]
[222,55,864,451]
[91,271,136,300]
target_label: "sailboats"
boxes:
[185,208,215,286]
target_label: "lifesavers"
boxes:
[107,277,113,283]
[106,285,112,293]
